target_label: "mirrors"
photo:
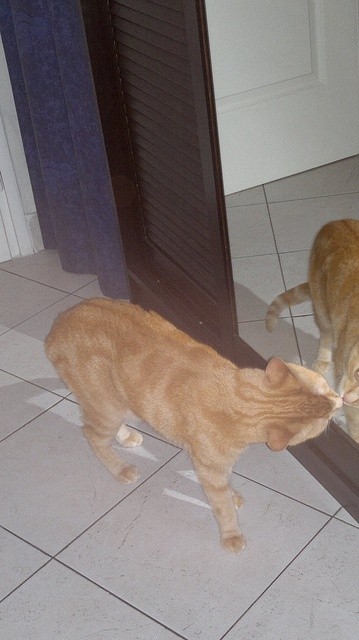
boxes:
[201,0,359,523]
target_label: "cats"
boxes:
[266,218,359,406]
[44,297,343,555]
